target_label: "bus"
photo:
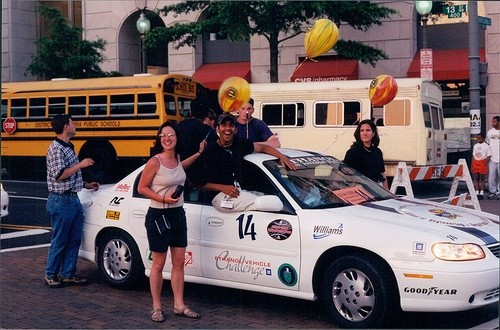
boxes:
[244,78,448,177]
[0,73,207,185]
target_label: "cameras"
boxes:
[171,184,184,199]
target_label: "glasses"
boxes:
[159,132,175,138]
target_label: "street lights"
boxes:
[136,12,150,74]
[416,1,433,49]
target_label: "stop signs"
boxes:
[3,117,17,134]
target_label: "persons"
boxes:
[486,116,500,200]
[283,158,326,208]
[470,133,491,200]
[190,113,298,205]
[174,95,280,170]
[137,122,208,322]
[44,114,100,287]
[330,119,388,189]
[473,114,478,120]
[338,164,361,175]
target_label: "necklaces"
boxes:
[363,146,372,152]
[219,137,231,152]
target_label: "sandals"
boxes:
[172,304,200,319]
[151,307,166,322]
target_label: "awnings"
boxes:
[290,55,358,82]
[407,48,485,81]
[192,62,251,90]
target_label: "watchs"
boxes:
[83,182,86,188]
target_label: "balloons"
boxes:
[304,19,339,58]
[217,76,251,113]
[369,74,398,106]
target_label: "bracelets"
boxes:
[199,152,201,154]
[163,196,169,209]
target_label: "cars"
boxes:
[77,147,500,330]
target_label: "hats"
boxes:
[216,114,237,125]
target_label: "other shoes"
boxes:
[59,275,87,286]
[44,275,63,288]
[487,193,499,200]
[477,194,484,200]
